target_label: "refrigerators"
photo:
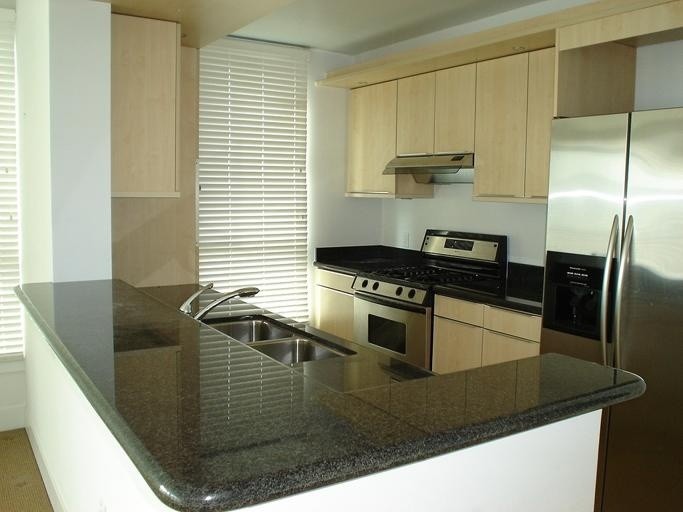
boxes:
[536,109,682,511]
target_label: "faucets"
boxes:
[179,281,260,322]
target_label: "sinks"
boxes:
[207,320,294,344]
[249,338,345,367]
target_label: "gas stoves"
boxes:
[350,264,481,308]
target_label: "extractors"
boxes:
[381,155,474,184]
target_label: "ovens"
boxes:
[353,288,432,370]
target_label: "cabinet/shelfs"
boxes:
[429,293,543,376]
[111,338,184,455]
[342,78,434,202]
[114,283,201,463]
[342,355,539,436]
[110,10,183,200]
[395,62,476,159]
[111,42,202,288]
[312,263,356,342]
[471,32,638,205]
